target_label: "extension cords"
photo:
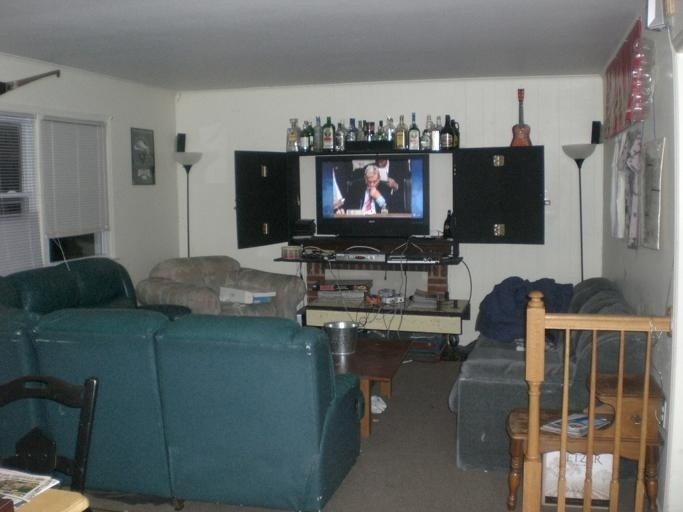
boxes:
[382,297,405,304]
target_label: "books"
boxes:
[219,290,273,305]
[540,412,611,438]
[220,285,277,298]
[408,288,439,309]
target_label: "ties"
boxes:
[362,189,371,213]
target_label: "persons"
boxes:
[361,159,404,213]
[334,165,394,215]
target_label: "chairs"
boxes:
[0,374,99,493]
[135,255,305,321]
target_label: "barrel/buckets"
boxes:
[324,321,359,354]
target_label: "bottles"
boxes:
[285,110,460,152]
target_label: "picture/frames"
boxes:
[130,128,156,186]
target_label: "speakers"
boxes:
[591,121,601,144]
[345,141,366,152]
[177,133,186,152]
[369,141,393,153]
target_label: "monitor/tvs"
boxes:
[315,155,430,239]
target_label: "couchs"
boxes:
[448,277,657,475]
[0,257,365,512]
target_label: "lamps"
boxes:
[171,152,203,258]
[562,144,596,281]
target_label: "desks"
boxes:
[505,371,665,512]
[14,487,89,512]
[301,298,469,335]
[274,236,463,265]
